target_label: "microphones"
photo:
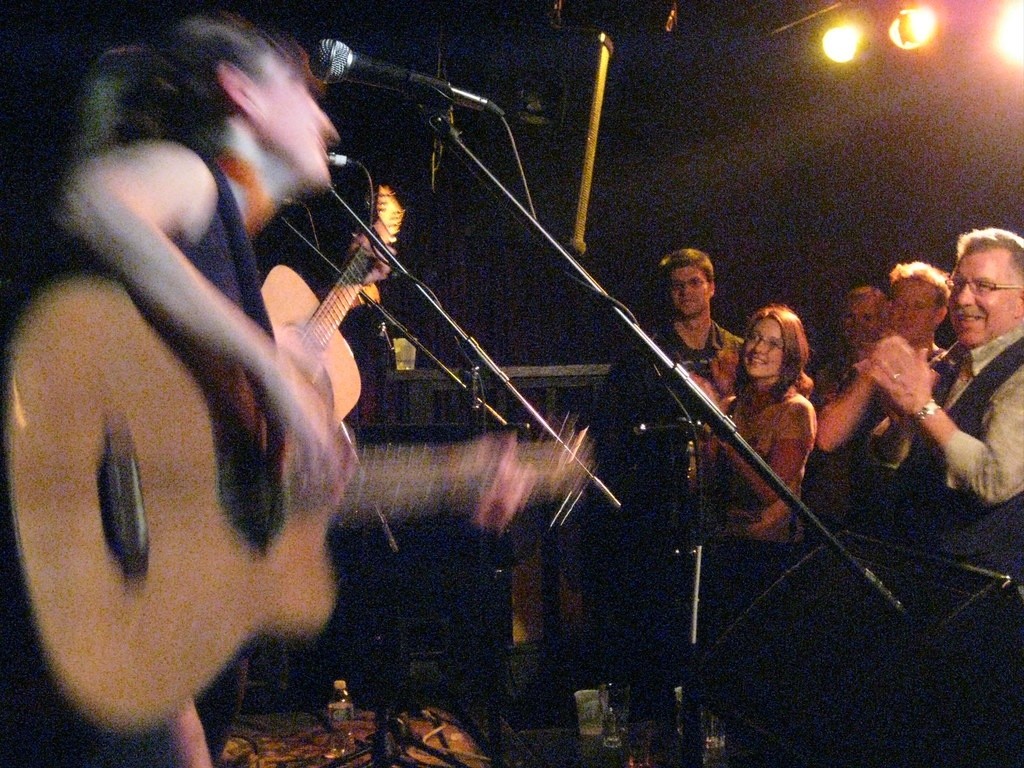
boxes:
[306,38,507,124]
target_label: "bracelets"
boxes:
[914,399,941,425]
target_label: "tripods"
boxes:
[319,328,465,768]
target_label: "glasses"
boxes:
[945,276,1024,297]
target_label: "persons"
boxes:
[63,11,533,768]
[607,249,817,541]
[816,226,1024,504]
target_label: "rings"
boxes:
[894,374,900,378]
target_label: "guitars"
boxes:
[259,187,409,427]
[0,272,599,737]
